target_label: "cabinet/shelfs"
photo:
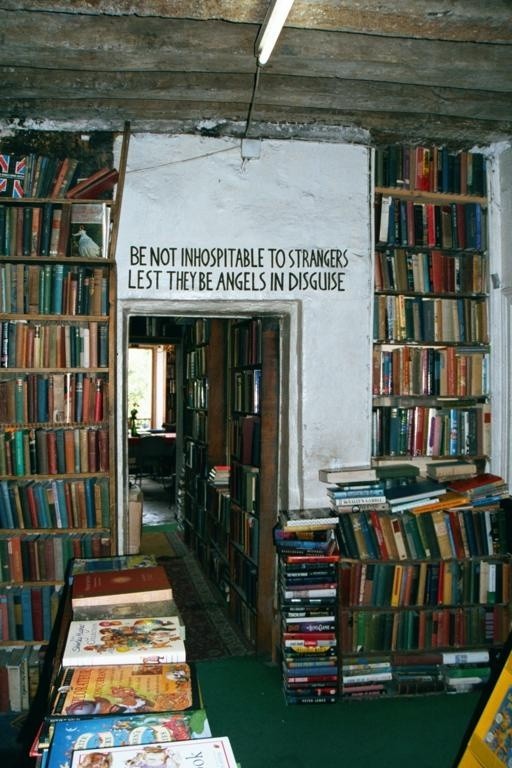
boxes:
[178,317,280,663]
[335,500,511,705]
[371,186,491,463]
[0,194,120,718]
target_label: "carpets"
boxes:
[140,522,255,663]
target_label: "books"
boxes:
[1,478,111,582]
[273,466,512,705]
[1,373,109,476]
[1,263,108,369]
[127,316,262,646]
[1,586,62,711]
[373,146,489,476]
[457,653,511,767]
[29,552,236,767]
[1,154,119,259]
